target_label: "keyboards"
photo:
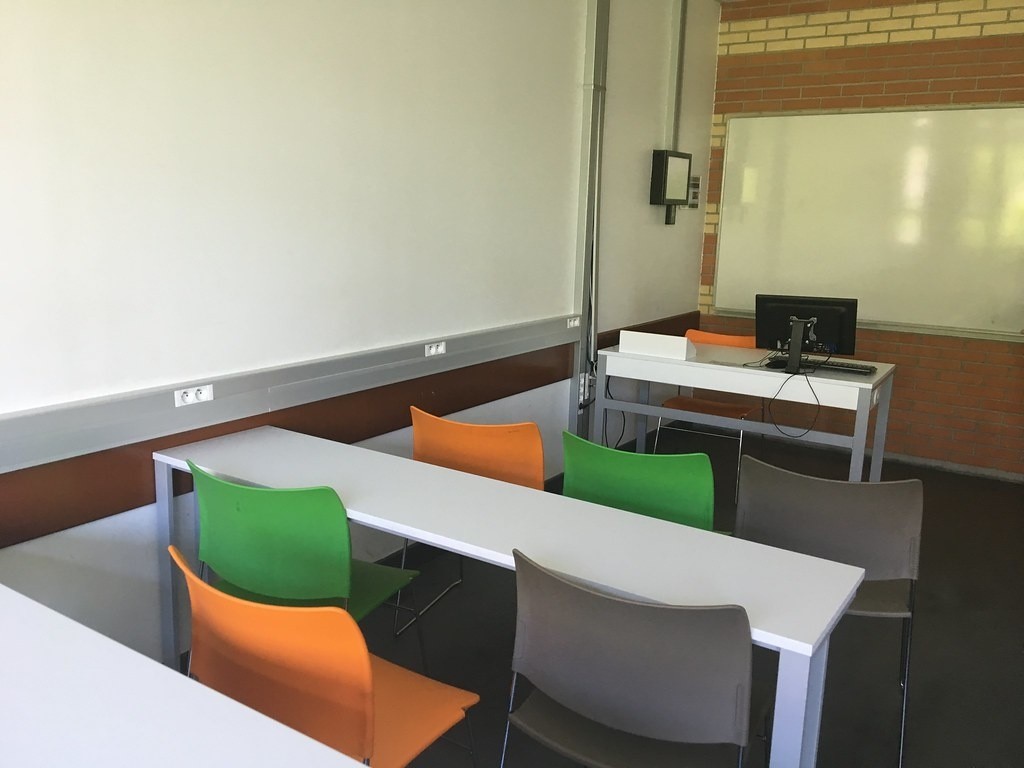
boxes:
[769,354,878,373]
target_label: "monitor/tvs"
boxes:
[756,295,858,374]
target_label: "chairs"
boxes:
[186,457,431,680]
[734,455,923,768]
[653,329,757,502]
[392,406,544,637]
[563,431,732,536]
[499,548,770,768]
[168,544,480,768]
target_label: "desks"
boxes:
[594,342,897,483]
[0,584,366,768]
[153,425,866,768]
